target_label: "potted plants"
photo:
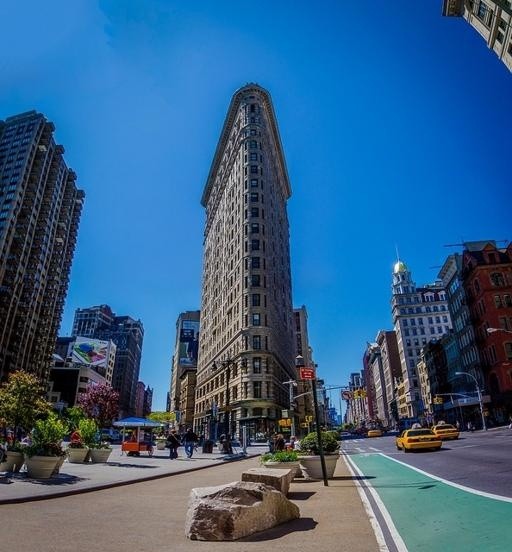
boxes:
[0,366,118,481]
[298,430,340,481]
[260,449,302,482]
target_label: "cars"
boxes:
[96,426,155,444]
[396,423,442,451]
[5,425,25,438]
[431,420,459,441]
[339,431,352,438]
[367,428,382,437]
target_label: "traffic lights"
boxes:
[278,420,281,425]
[353,391,355,399]
[357,391,359,396]
[363,390,366,399]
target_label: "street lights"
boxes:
[307,345,329,487]
[295,353,309,438]
[484,326,511,337]
[209,350,248,455]
[455,370,487,431]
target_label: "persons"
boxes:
[456,419,460,432]
[274,432,284,453]
[168,428,179,459]
[467,418,474,431]
[290,432,297,449]
[181,428,197,456]
